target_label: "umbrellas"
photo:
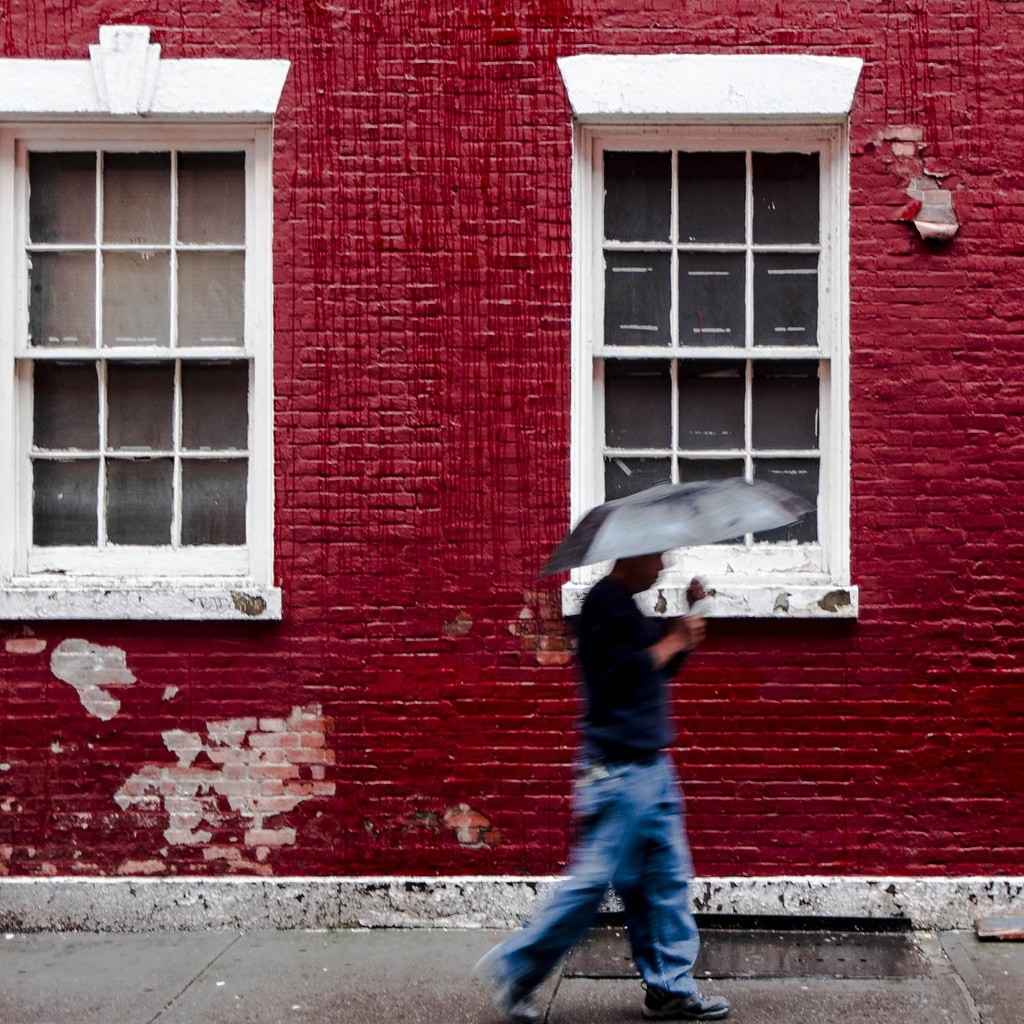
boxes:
[542,478,816,578]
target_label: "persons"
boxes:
[477,552,731,1023]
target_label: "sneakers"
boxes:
[639,980,732,1021]
[472,956,543,1024]
